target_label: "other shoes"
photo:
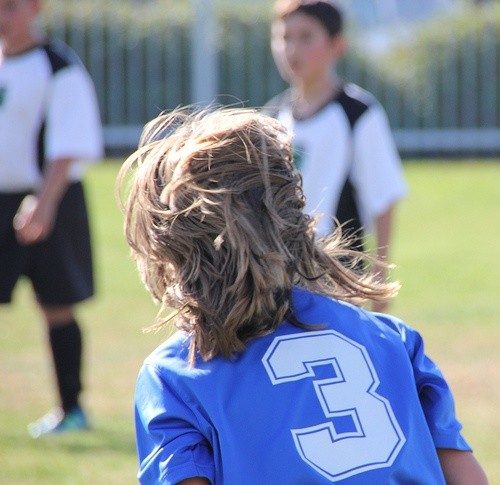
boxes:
[23,406,86,439]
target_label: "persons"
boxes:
[266,0,406,317]
[116,103,491,484]
[0,0,107,435]
